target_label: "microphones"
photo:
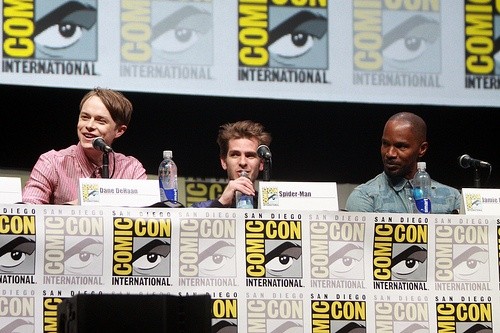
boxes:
[92,137,113,153]
[256,144,272,159]
[457,154,491,169]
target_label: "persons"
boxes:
[23,87,148,210]
[191,119,272,208]
[344,111,462,215]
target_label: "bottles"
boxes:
[157,151,179,207]
[413,162,431,213]
[235,172,254,209]
